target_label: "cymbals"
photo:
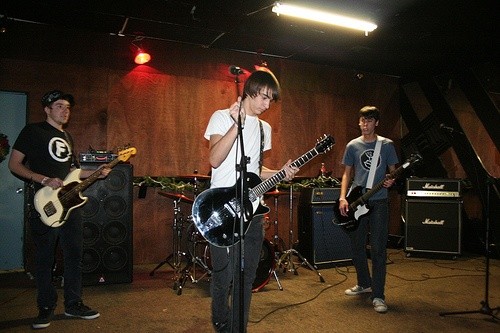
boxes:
[280,176,309,184]
[157,191,194,205]
[176,173,211,180]
[263,191,289,196]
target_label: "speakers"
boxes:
[78,162,135,286]
[297,187,356,268]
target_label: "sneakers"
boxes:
[64,296,101,320]
[32,304,57,327]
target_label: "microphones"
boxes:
[438,124,463,135]
[228,65,243,75]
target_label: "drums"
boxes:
[203,238,277,292]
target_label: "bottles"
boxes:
[320,163,326,177]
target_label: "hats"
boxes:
[41,89,75,108]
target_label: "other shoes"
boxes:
[345,285,372,295]
[373,297,388,312]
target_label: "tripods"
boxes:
[147,183,326,296]
[437,134,500,322]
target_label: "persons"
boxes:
[203,67,300,333]
[338,106,398,310]
[7,89,112,328]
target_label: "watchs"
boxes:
[41,176,50,185]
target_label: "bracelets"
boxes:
[339,197,346,202]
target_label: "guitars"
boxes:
[332,153,423,234]
[34,147,137,229]
[191,134,335,249]
